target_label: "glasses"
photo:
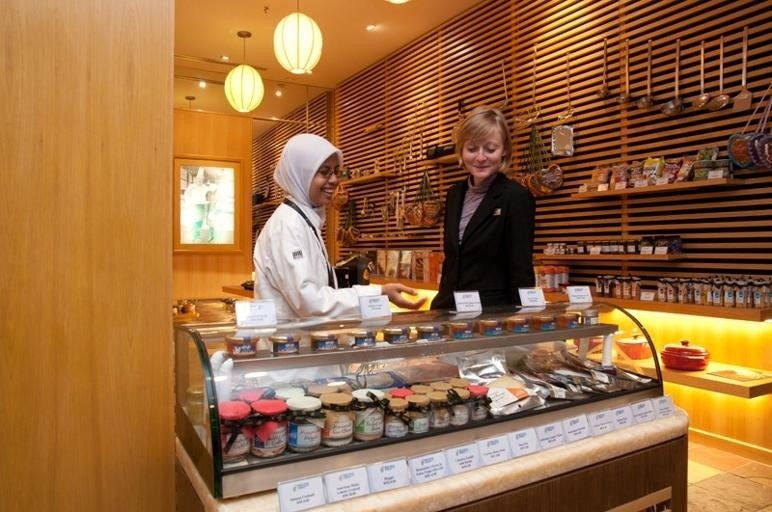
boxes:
[317,167,343,178]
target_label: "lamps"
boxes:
[223,0,324,113]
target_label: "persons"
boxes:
[423,107,540,316]
[182,170,217,244]
[245,132,431,383]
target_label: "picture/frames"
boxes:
[172,152,245,255]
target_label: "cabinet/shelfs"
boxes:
[175,303,691,512]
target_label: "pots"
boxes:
[660,338,710,371]
[616,334,653,360]
